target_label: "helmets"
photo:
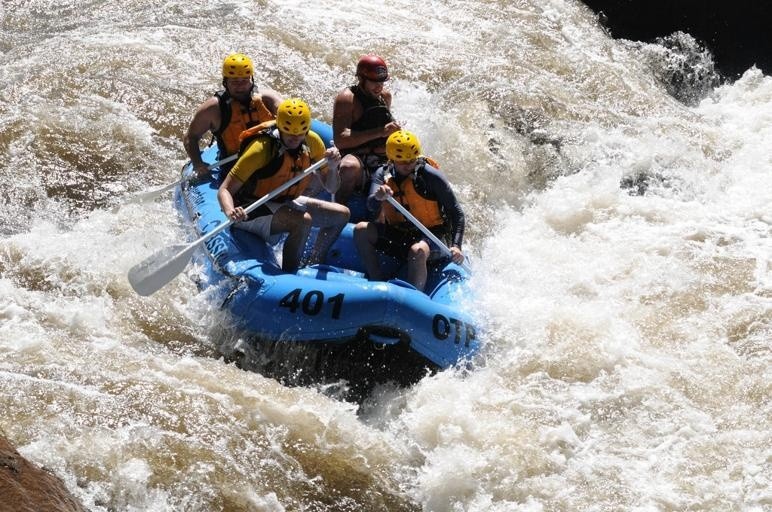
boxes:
[275,98,311,136]
[355,55,390,81]
[385,130,422,161]
[223,53,254,79]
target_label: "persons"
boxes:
[217,97,352,276]
[331,56,402,207]
[183,52,284,188]
[353,130,465,294]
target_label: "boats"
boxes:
[174,114,491,387]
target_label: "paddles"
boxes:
[127,155,331,297]
[110,151,241,214]
